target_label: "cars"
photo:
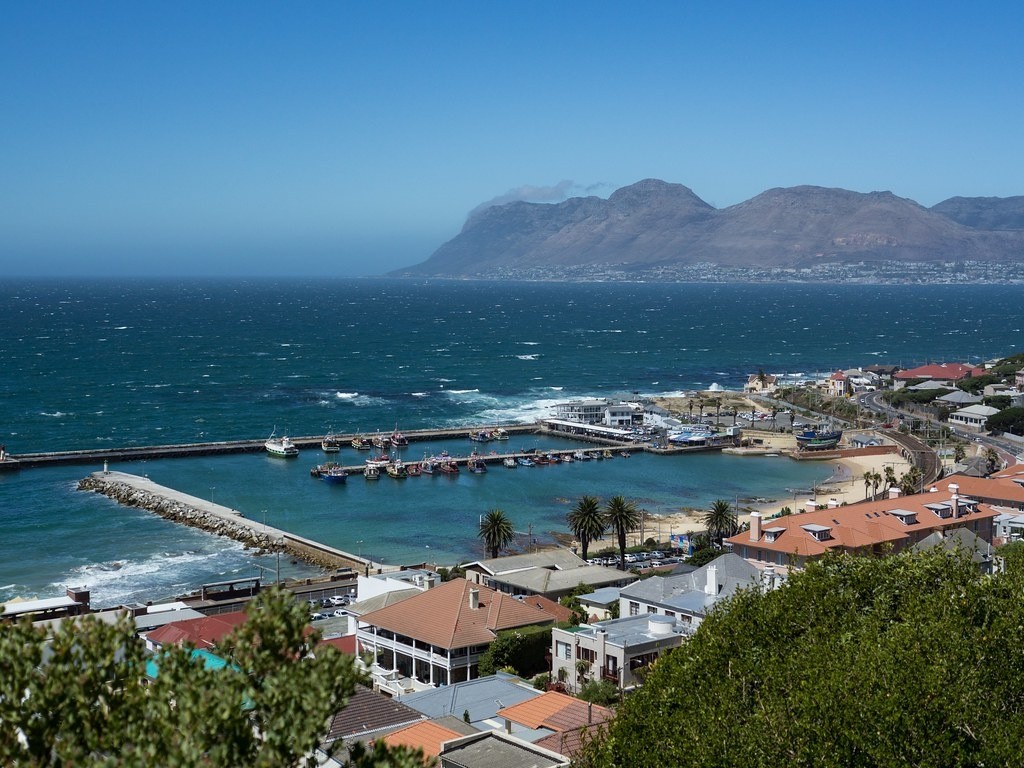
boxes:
[595,408,803,452]
[300,593,357,623]
[580,550,691,572]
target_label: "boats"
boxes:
[351,432,372,449]
[469,428,491,442]
[365,465,381,480]
[419,450,435,474]
[263,425,298,457]
[315,461,348,481]
[492,424,509,441]
[385,452,407,478]
[366,454,392,466]
[390,420,408,446]
[371,428,390,449]
[321,425,342,451]
[438,447,632,476]
[408,465,420,477]
